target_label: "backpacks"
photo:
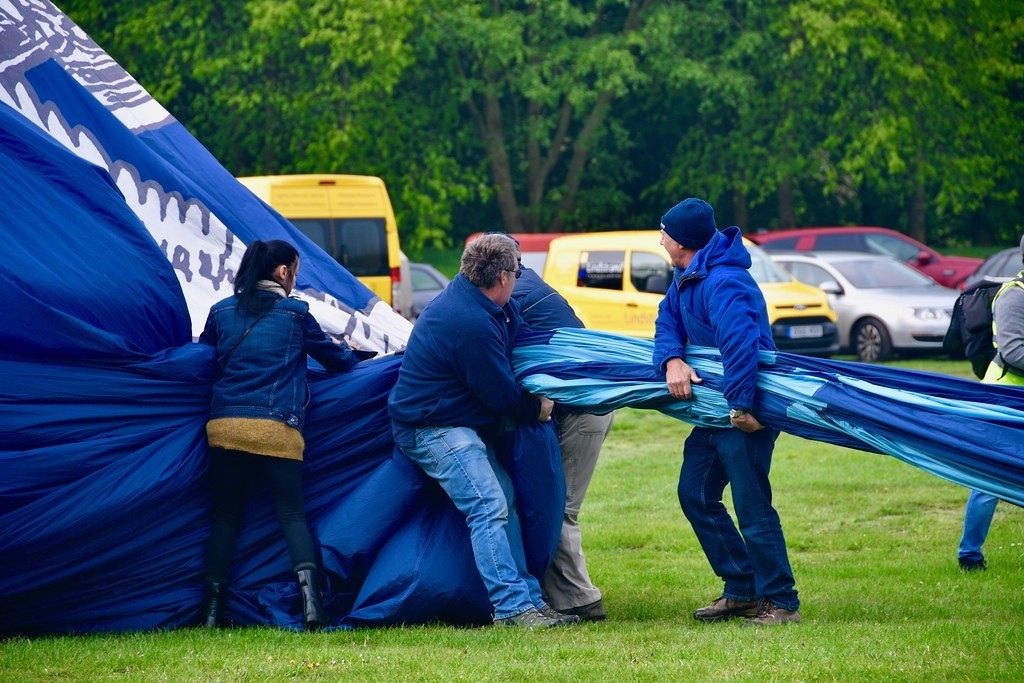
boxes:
[942,275,1024,380]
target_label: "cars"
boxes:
[768,251,963,363]
[408,264,451,319]
[957,247,1023,296]
[742,225,987,290]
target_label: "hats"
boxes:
[660,199,716,251]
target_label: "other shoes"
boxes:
[959,559,988,571]
[558,600,607,621]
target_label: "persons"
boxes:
[959,236,1024,571]
[198,240,378,628]
[337,244,349,267]
[652,198,801,630]
[388,233,614,631]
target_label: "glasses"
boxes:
[495,269,522,279]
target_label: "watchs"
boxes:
[729,409,746,418]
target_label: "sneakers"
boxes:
[494,608,565,629]
[693,597,773,623]
[538,605,581,625]
[740,596,801,628]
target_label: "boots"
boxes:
[293,562,328,631]
[200,578,229,630]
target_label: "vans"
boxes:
[542,229,841,360]
[465,232,569,281]
[234,173,412,323]
